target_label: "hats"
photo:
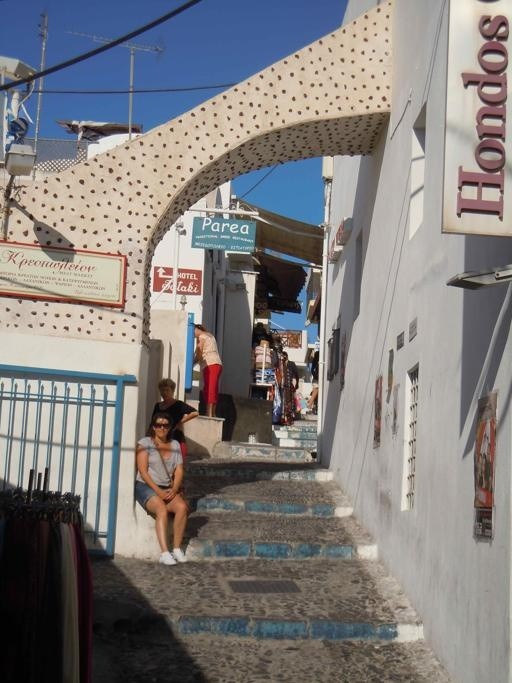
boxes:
[256,340,270,352]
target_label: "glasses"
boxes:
[153,423,170,429]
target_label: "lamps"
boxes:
[3,144,36,197]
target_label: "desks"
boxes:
[249,383,273,400]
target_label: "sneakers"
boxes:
[173,548,188,563]
[159,552,177,565]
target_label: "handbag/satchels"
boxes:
[271,330,280,342]
[170,478,184,495]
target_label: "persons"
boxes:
[475,433,493,507]
[135,413,190,565]
[146,379,199,470]
[251,340,283,425]
[194,324,223,416]
[308,350,318,410]
[283,351,299,390]
